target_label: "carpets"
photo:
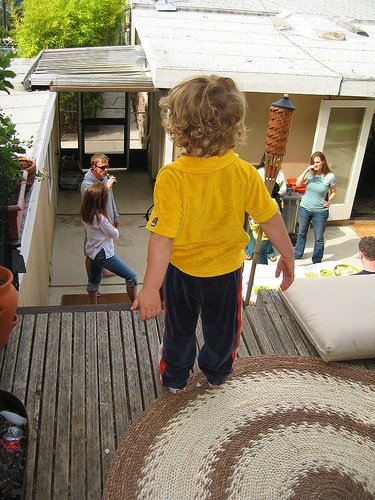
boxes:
[61,293,131,305]
[102,354,375,500]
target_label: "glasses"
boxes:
[95,164,111,170]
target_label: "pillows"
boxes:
[278,274,375,364]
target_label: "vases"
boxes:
[0,265,24,347]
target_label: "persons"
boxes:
[79,153,138,304]
[245,151,337,266]
[130,74,295,395]
[347,236,375,275]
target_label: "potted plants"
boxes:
[0,49,36,239]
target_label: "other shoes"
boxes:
[269,255,277,262]
[206,381,223,390]
[166,386,187,395]
[245,254,252,261]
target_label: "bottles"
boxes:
[105,172,120,188]
[278,180,284,188]
[323,192,329,208]
